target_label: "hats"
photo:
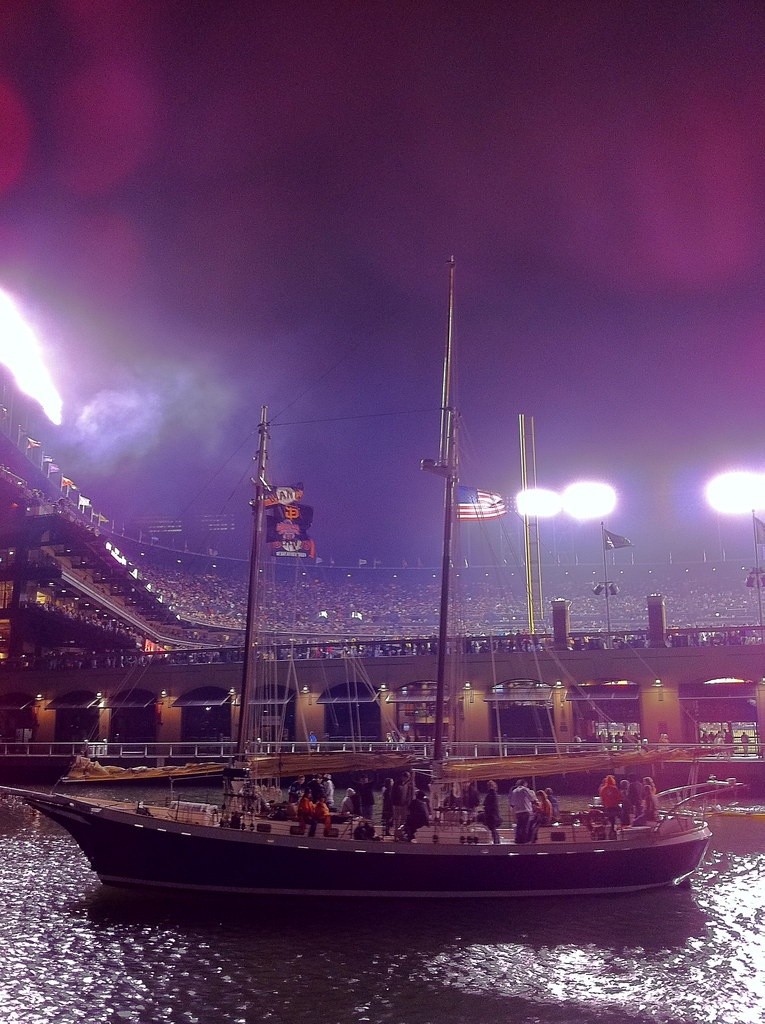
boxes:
[361,774,368,778]
[401,771,410,778]
[324,774,331,779]
[383,778,393,783]
[345,788,356,797]
[415,790,427,799]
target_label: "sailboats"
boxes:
[0,249,752,894]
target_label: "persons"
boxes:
[0,550,765,851]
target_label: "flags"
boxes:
[80,495,90,506]
[42,455,53,463]
[26,437,41,449]
[753,517,765,545]
[263,481,304,508]
[49,463,59,473]
[61,477,73,486]
[265,504,315,527]
[271,540,315,559]
[603,529,634,550]
[86,505,92,508]
[456,485,508,522]
[266,526,309,543]
[93,509,98,518]
[100,514,109,523]
[69,484,77,490]
